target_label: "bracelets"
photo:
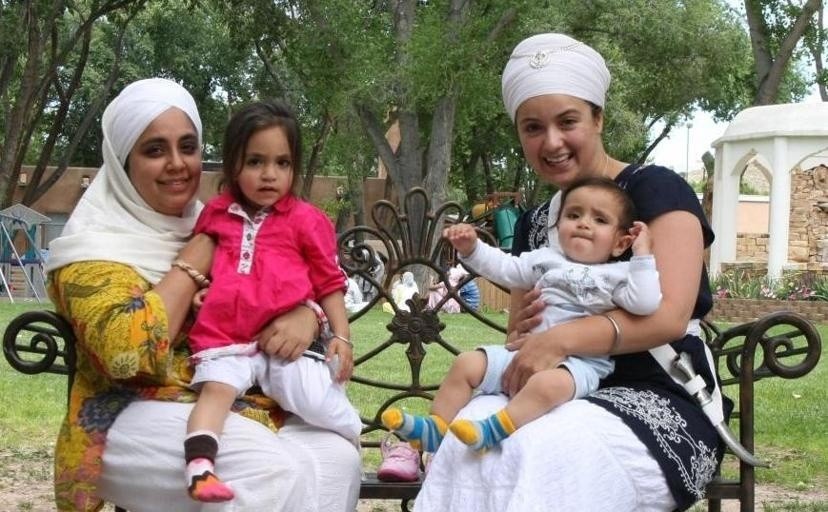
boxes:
[302,299,331,341]
[172,260,210,287]
[326,331,357,348]
[598,310,623,358]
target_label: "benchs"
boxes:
[3,185,821,512]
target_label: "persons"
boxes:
[343,272,369,315]
[409,32,734,512]
[380,265,484,320]
[378,176,663,459]
[355,238,383,300]
[40,77,364,512]
[178,98,362,502]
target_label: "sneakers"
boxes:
[378,430,422,480]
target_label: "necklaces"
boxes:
[600,153,614,181]
